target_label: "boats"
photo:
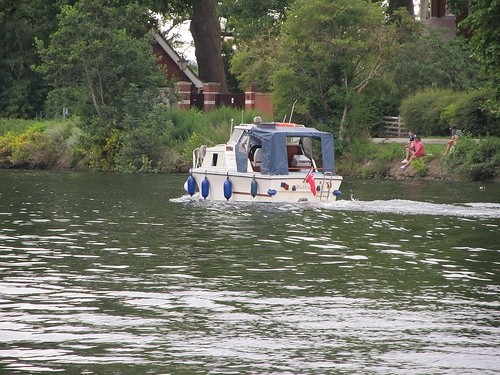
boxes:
[182,98,344,205]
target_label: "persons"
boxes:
[248,115,263,162]
[400,135,424,169]
[443,126,463,157]
[404,131,418,151]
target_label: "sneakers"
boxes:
[402,159,409,163]
[400,165,405,169]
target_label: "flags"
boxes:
[305,166,317,197]
